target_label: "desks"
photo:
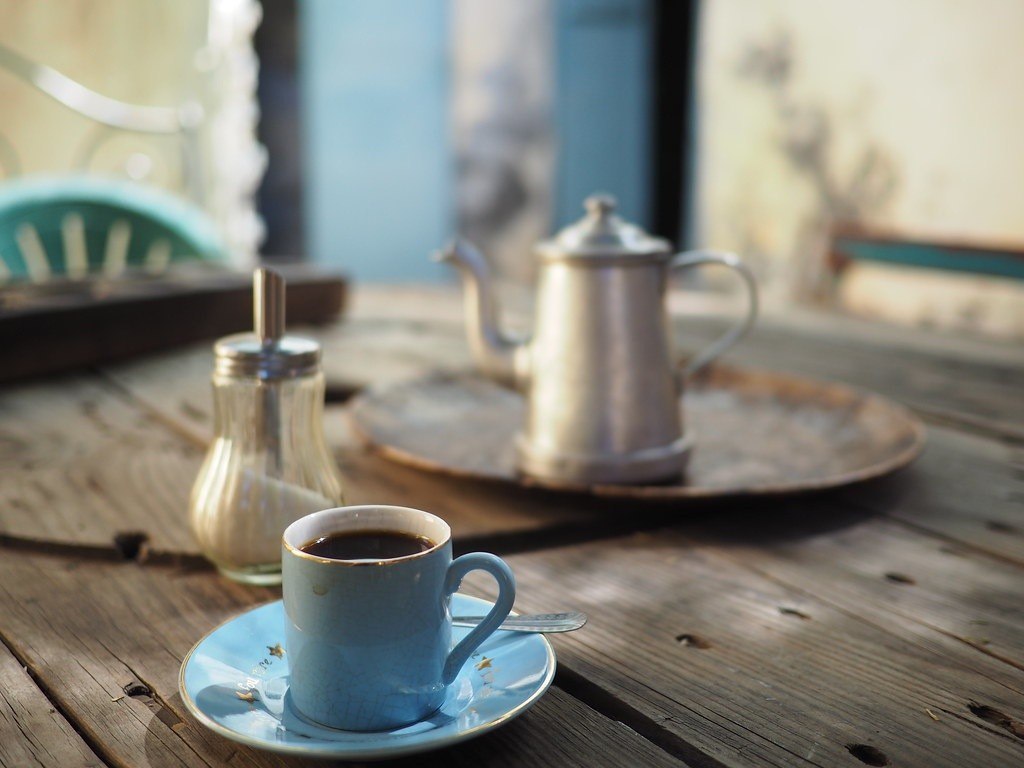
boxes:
[0,285,1024,767]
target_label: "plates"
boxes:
[179,589,556,759]
[347,359,927,501]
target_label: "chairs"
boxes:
[781,205,1024,303]
[0,178,220,283]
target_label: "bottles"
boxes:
[191,268,342,585]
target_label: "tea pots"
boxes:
[429,192,758,483]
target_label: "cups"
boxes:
[281,505,515,733]
